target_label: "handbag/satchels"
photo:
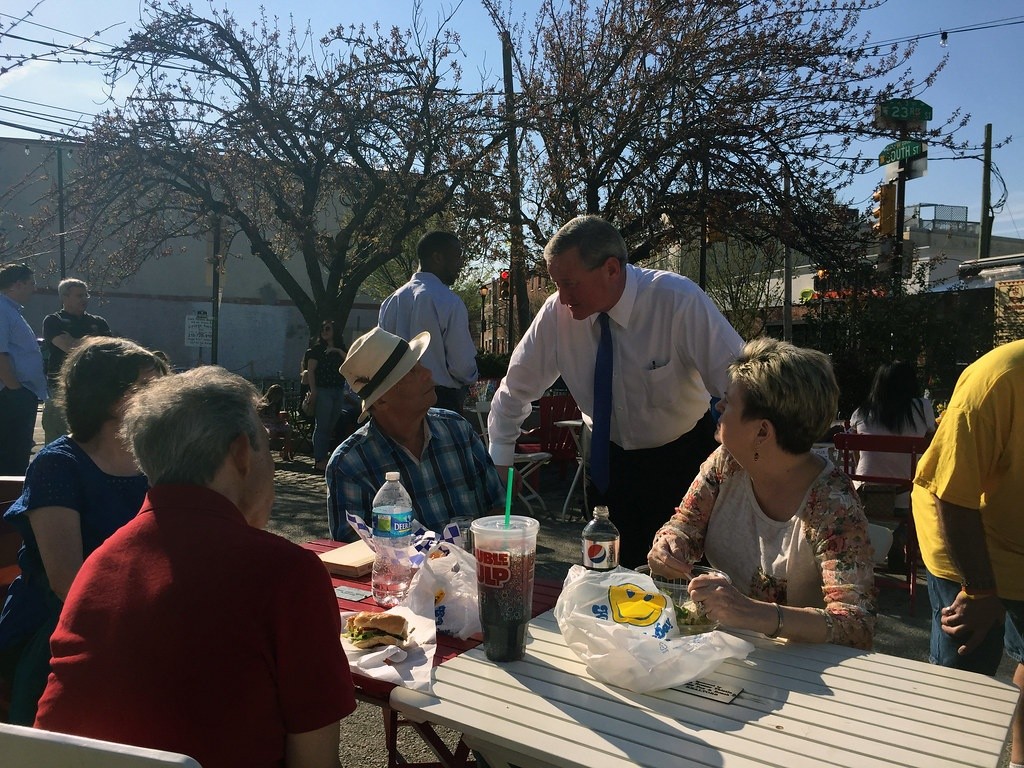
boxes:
[553,563,755,695]
[302,391,316,416]
[406,540,479,639]
[852,483,910,522]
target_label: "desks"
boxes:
[297,540,563,767]
[389,606,1021,768]
[554,420,586,522]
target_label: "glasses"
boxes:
[320,325,333,332]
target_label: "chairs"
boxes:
[476,401,552,516]
[832,432,931,617]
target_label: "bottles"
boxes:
[580,505,621,572]
[371,472,413,609]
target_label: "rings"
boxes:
[946,607,953,616]
[705,614,718,630]
[698,604,706,614]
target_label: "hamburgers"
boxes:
[345,611,409,648]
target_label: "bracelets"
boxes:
[961,576,999,600]
[764,602,786,638]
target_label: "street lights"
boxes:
[478,284,490,352]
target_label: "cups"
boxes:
[468,514,540,663]
[449,516,474,556]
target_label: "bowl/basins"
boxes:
[633,564,732,636]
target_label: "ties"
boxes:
[590,315,613,497]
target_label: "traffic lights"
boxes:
[871,181,895,239]
[704,202,726,243]
[498,268,509,300]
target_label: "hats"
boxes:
[339,327,431,424]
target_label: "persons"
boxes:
[257,384,293,460]
[910,338,1024,768]
[40,276,111,446]
[647,337,876,653]
[0,333,172,694]
[304,320,361,473]
[0,264,48,476]
[34,363,358,768]
[485,215,750,567]
[849,359,937,573]
[328,326,512,542]
[378,230,479,418]
